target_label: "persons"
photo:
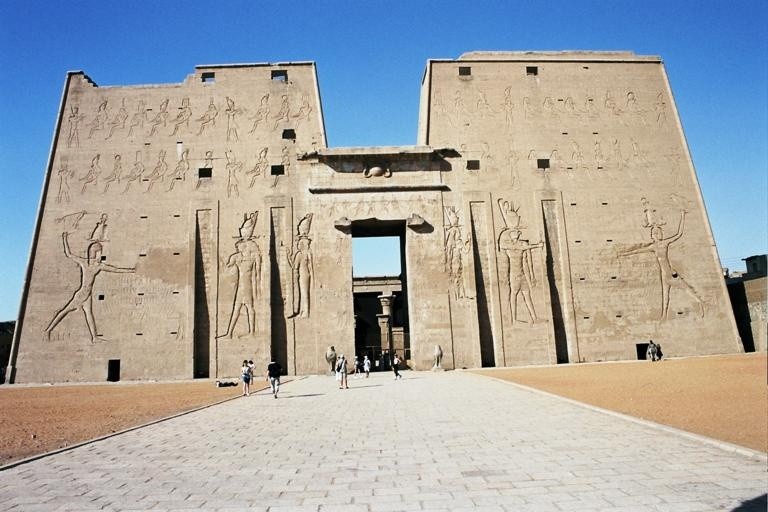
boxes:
[248,361,256,384]
[392,354,402,381]
[335,355,349,389]
[363,356,371,378]
[240,360,251,398]
[353,356,361,376]
[647,340,663,361]
[265,358,282,399]
